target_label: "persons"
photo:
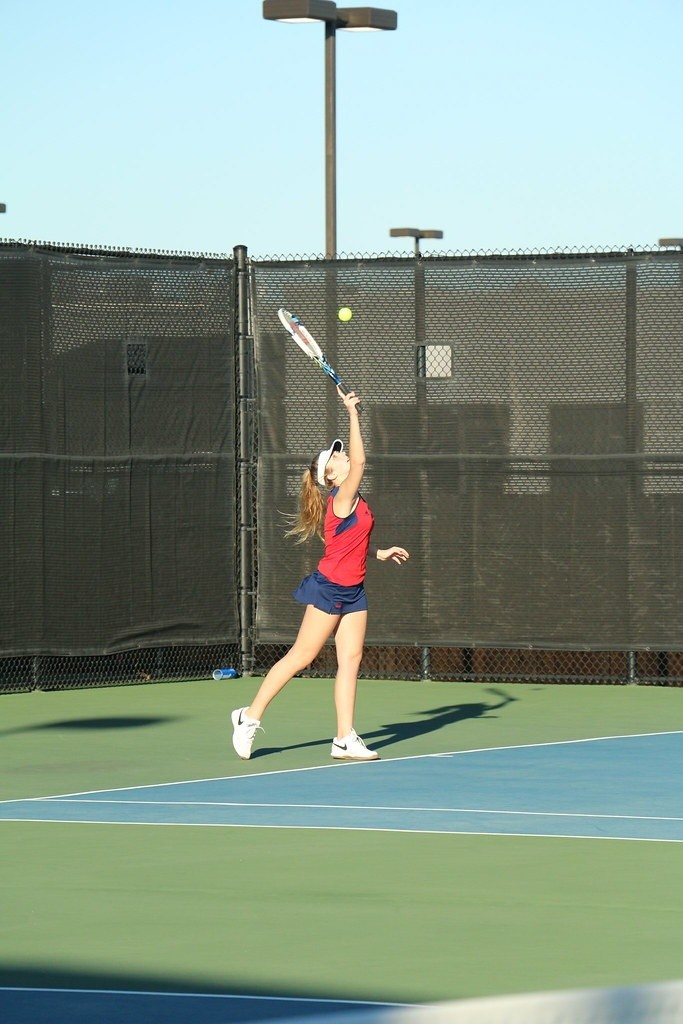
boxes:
[231,384,409,761]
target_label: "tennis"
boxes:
[338,308,353,322]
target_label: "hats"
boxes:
[317,439,343,486]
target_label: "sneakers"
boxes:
[231,706,265,760]
[332,728,378,759]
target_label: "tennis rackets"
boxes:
[277,307,364,415]
